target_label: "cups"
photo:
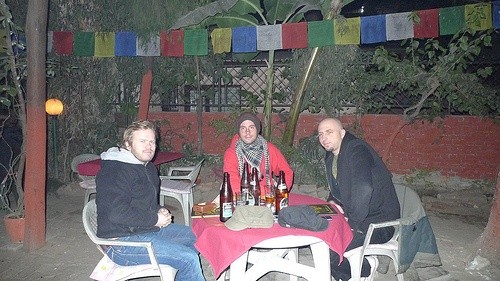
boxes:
[264,184,274,205]
[236,191,247,206]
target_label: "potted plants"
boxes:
[0,0,77,244]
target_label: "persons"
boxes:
[95,120,207,281]
[317,118,400,281]
[223,112,295,195]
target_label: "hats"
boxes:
[224,205,273,231]
[236,112,260,133]
[278,205,329,231]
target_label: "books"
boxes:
[307,204,338,215]
[190,206,220,219]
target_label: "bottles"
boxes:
[275,171,288,215]
[240,162,250,205]
[219,172,233,222]
[250,167,260,206]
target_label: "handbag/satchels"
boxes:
[193,202,216,213]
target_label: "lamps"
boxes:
[45,99,64,182]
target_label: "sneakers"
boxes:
[360,255,380,281]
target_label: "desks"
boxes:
[78,151,184,177]
[191,192,354,281]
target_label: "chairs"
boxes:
[331,183,442,281]
[82,199,180,281]
[71,154,101,208]
[159,158,206,227]
